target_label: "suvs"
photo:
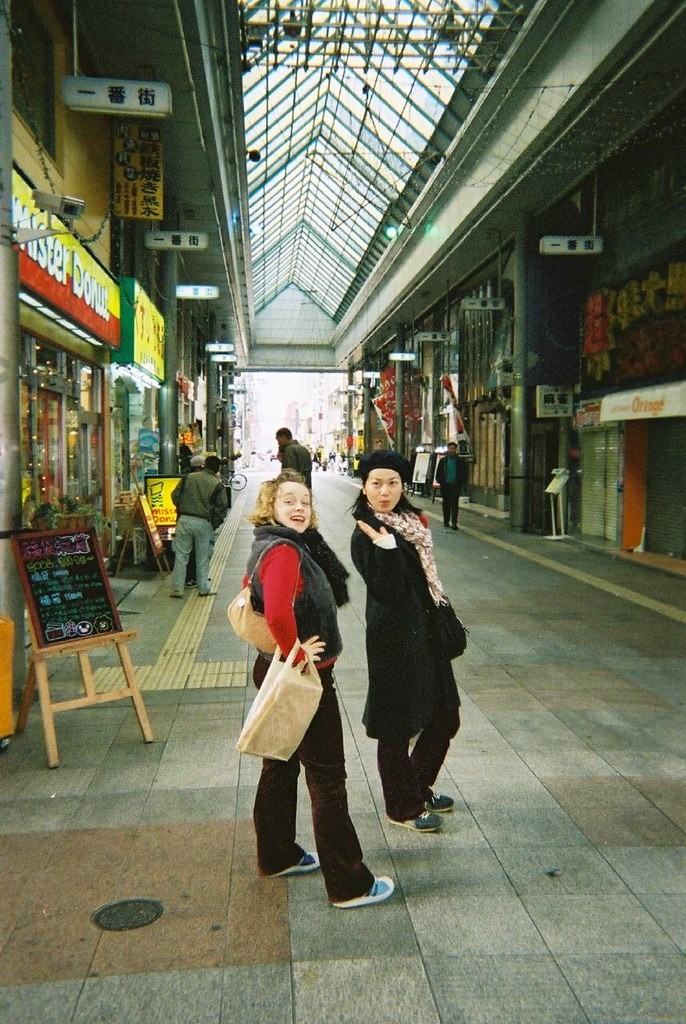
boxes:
[269,449,279,461]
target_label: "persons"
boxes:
[436,442,467,529]
[169,455,227,597]
[276,428,311,488]
[184,456,211,588]
[345,450,461,832]
[309,447,336,471]
[242,469,394,908]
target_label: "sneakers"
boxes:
[331,876,394,908]
[265,851,321,879]
[422,792,454,812]
[387,812,443,831]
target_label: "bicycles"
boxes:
[215,469,247,491]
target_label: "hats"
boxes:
[358,449,413,484]
[190,455,206,467]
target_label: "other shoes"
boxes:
[444,522,450,531]
[199,589,218,597]
[452,522,458,529]
[168,590,183,598]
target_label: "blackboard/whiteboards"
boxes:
[11,524,126,653]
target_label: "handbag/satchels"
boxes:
[227,540,301,657]
[432,597,467,661]
[235,637,323,761]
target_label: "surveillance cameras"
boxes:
[32,189,85,219]
